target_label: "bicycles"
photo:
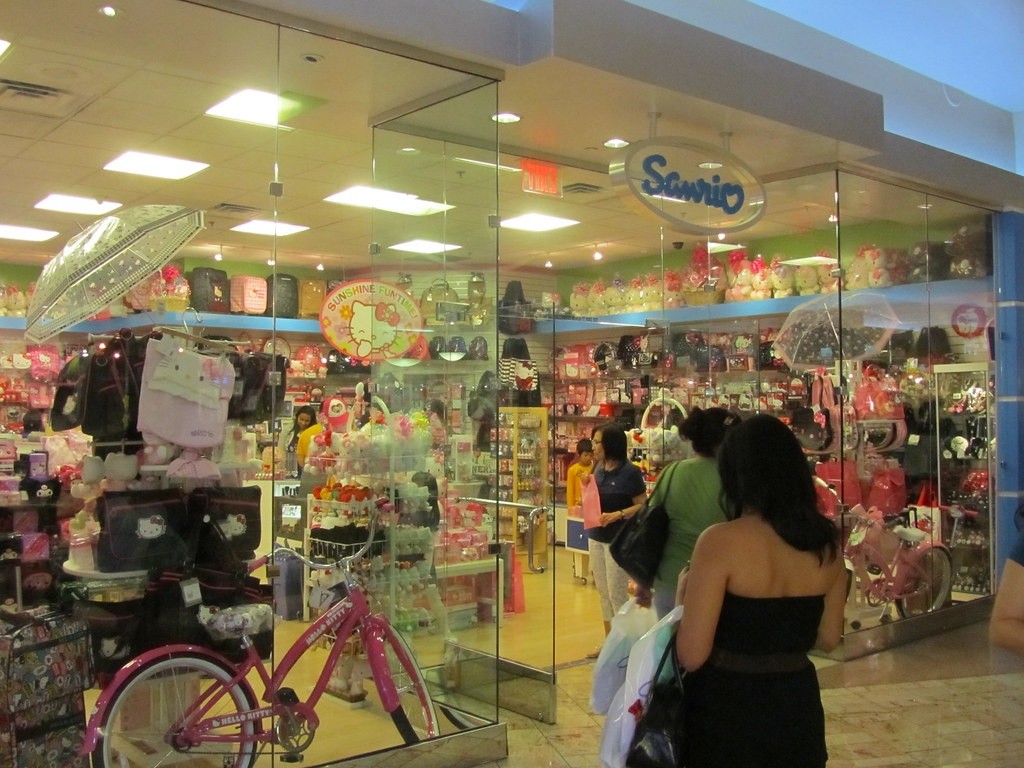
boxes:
[82,497,441,768]
[811,475,954,619]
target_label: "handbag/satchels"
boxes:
[15,302,289,680]
[580,473,604,530]
[599,604,684,767]
[186,264,541,446]
[589,597,658,716]
[608,460,680,591]
[544,323,956,516]
[627,630,693,768]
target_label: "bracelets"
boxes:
[620,509,625,519]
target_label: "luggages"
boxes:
[0,533,98,768]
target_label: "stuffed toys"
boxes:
[71,430,256,514]
[306,431,450,703]
[540,227,992,316]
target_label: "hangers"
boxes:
[150,306,230,348]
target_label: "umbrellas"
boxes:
[771,286,901,377]
[24,206,208,351]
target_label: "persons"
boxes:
[23,409,50,434]
[396,471,458,659]
[467,369,496,500]
[283,404,319,480]
[650,407,729,623]
[581,425,647,659]
[674,415,846,768]
[566,439,594,578]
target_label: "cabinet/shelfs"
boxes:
[928,360,996,602]
[0,280,991,632]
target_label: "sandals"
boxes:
[585,639,606,658]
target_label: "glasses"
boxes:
[591,439,602,446]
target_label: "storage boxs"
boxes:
[437,603,478,631]
